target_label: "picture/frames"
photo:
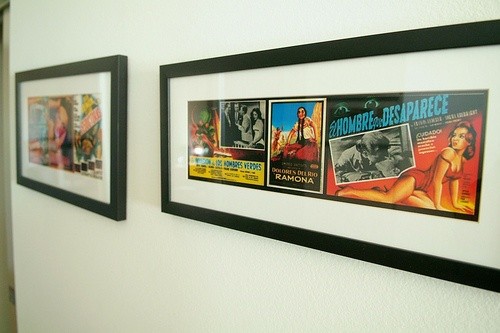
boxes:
[160,19,500,294]
[16,52,129,222]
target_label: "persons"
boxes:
[341,151,413,182]
[25,95,101,175]
[284,106,318,161]
[273,126,287,153]
[249,107,265,149]
[192,117,218,156]
[230,102,242,142]
[336,131,391,175]
[235,105,253,145]
[222,101,232,147]
